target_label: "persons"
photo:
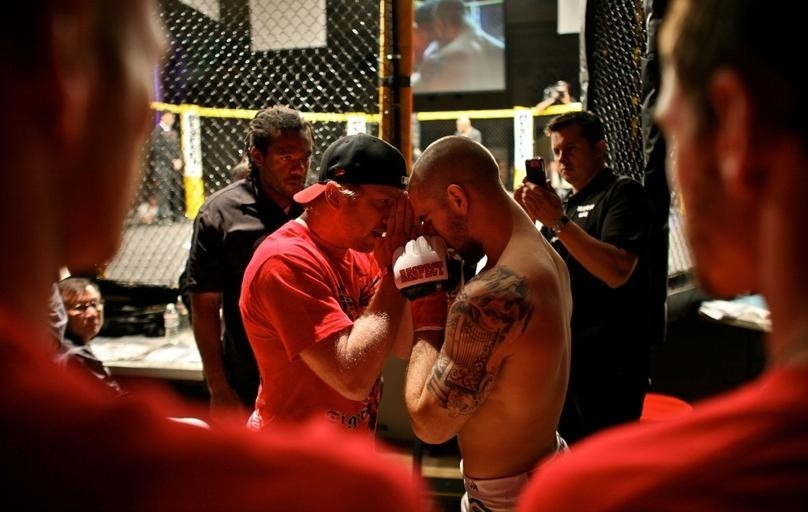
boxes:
[151,109,187,221]
[414,6,436,66]
[2,1,432,512]
[517,3,807,510]
[515,111,665,441]
[237,132,415,441]
[454,116,482,144]
[534,80,577,110]
[415,1,508,92]
[62,274,127,401]
[391,132,572,511]
[183,106,316,428]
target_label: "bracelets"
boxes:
[549,216,570,236]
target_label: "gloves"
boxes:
[390,235,476,300]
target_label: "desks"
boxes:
[88,334,211,405]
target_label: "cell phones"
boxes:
[525,158,546,187]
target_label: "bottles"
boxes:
[164,295,190,343]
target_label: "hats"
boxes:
[291,131,409,205]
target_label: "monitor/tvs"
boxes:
[411,0,513,112]
